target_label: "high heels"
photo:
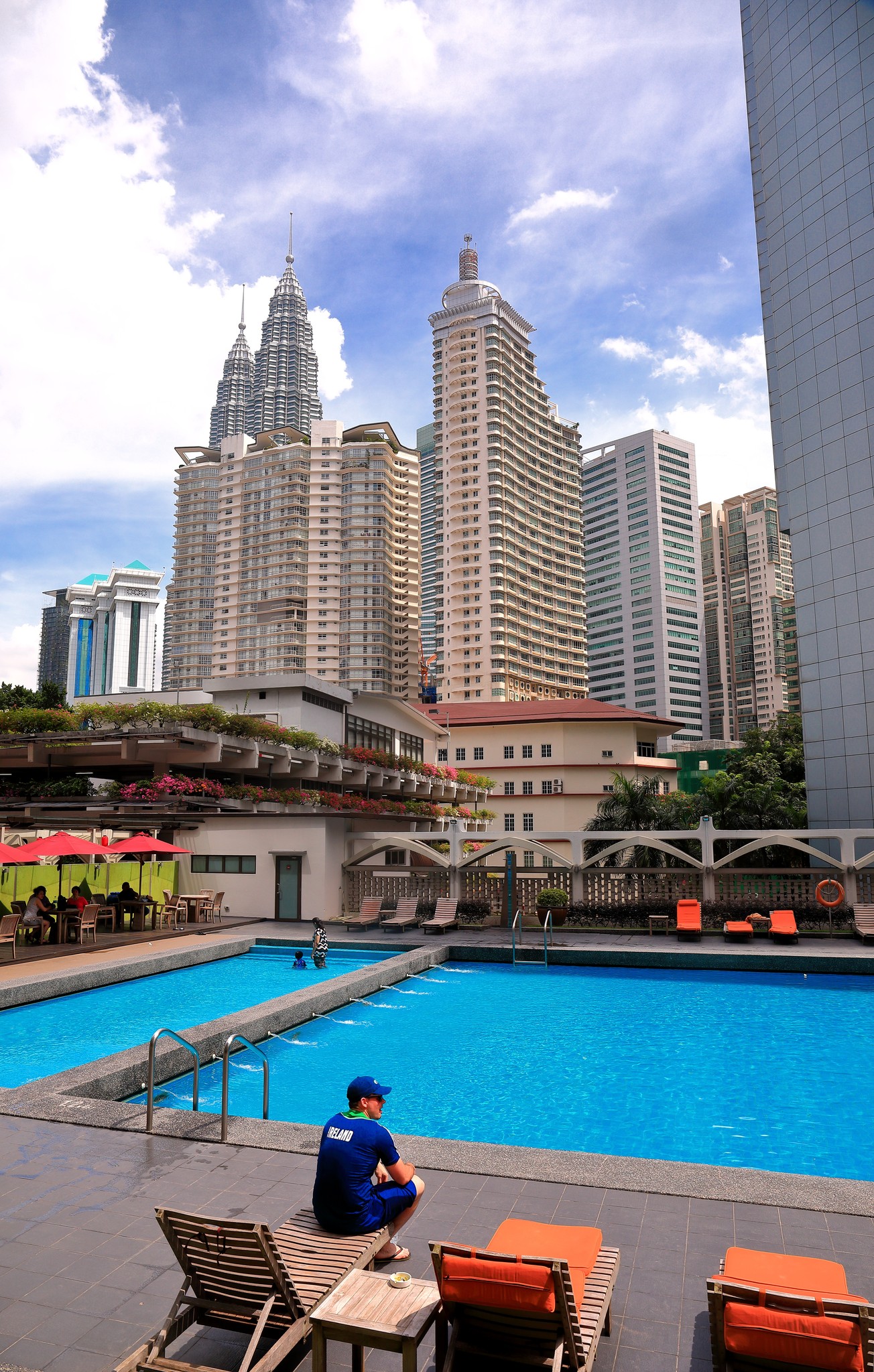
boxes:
[74,936,79,940]
[68,932,71,938]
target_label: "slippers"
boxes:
[194,931,202,934]
[374,1245,411,1263]
[179,928,185,930]
[173,928,179,930]
[199,932,206,935]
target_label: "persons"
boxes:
[313,1076,425,1262]
[292,951,306,967]
[62,886,88,942]
[23,885,56,944]
[310,917,328,968]
[118,882,150,922]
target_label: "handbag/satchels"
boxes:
[138,895,148,902]
[57,895,68,911]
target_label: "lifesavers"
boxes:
[815,879,845,908]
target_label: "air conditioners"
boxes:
[553,779,561,793]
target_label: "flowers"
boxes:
[431,841,490,852]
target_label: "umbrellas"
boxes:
[106,831,194,898]
[0,830,123,896]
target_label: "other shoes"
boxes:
[132,918,134,922]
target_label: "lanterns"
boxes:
[101,835,108,847]
[37,836,43,841]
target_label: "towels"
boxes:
[745,913,768,923]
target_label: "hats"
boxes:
[347,1076,392,1099]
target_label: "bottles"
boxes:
[53,896,57,909]
[106,892,121,925]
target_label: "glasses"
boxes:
[44,890,47,892]
[365,1094,383,1102]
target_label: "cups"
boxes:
[390,1233,398,1245]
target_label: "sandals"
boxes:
[38,938,50,944]
[26,933,34,943]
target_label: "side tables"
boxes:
[649,916,669,937]
[750,918,771,939]
[378,910,396,929]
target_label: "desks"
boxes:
[46,908,81,943]
[178,895,210,923]
[307,1269,447,1372]
[120,901,158,931]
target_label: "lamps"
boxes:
[95,865,101,869]
[450,820,457,824]
[158,863,163,866]
[702,816,710,822]
[452,866,852,874]
[2,868,9,873]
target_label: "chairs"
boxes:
[723,921,754,942]
[852,904,874,945]
[112,1206,873,1372]
[676,900,701,941]
[344,896,462,935]
[0,889,226,959]
[769,910,799,944]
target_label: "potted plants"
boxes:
[537,889,569,926]
